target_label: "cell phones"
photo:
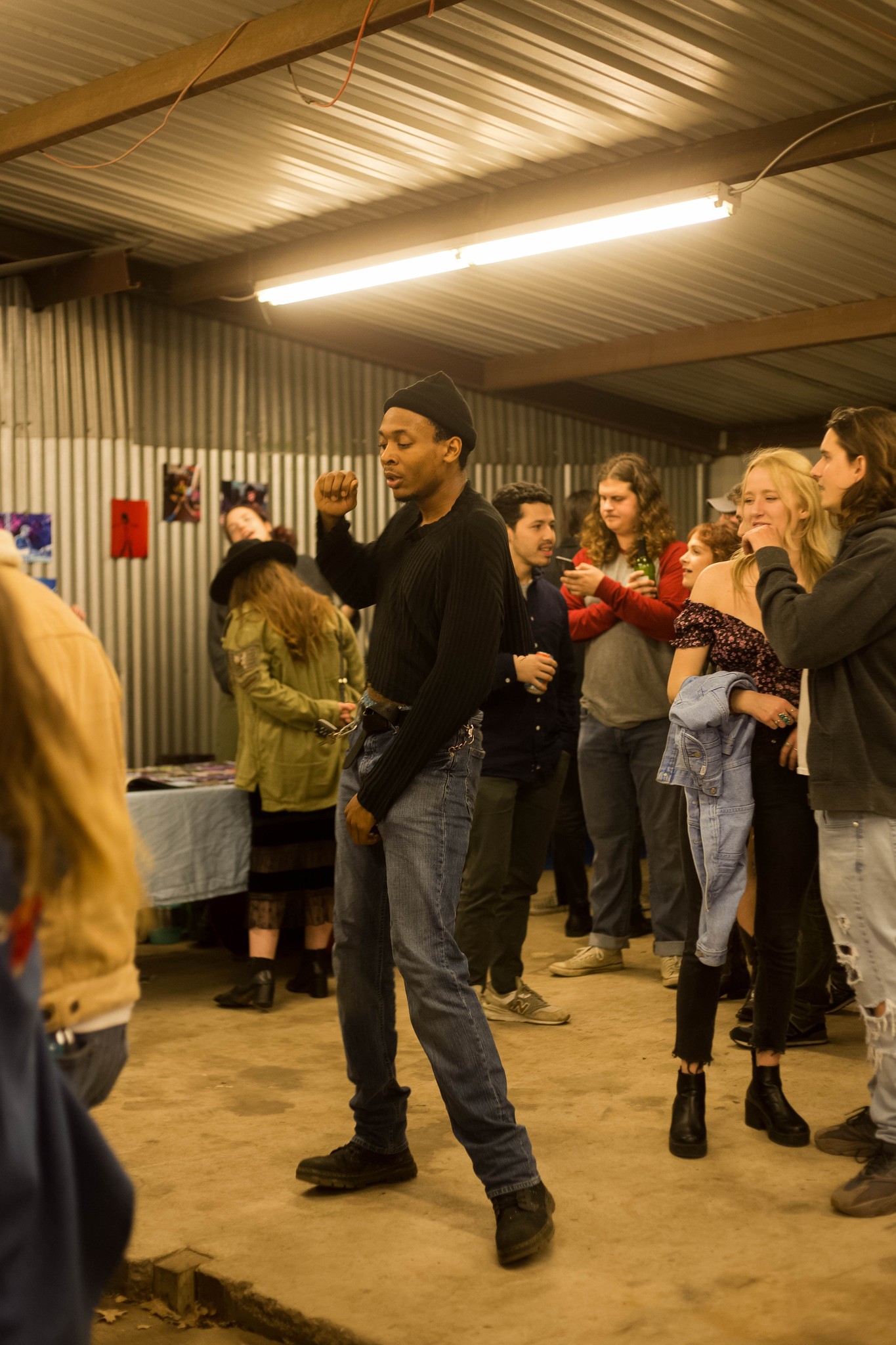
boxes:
[556,556,575,576]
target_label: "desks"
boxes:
[115,760,257,910]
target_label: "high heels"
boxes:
[213,969,275,1008]
[287,961,328,998]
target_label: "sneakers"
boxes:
[479,984,570,1025]
[815,1106,882,1157]
[826,985,859,1014]
[549,946,623,977]
[831,1141,896,1217]
[729,1014,828,1048]
[661,956,683,987]
[491,1180,554,1264]
[297,1142,417,1188]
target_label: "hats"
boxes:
[707,483,743,513]
[210,539,297,605]
[383,371,477,450]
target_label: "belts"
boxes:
[340,701,399,769]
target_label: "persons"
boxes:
[296,372,554,1266]
[551,451,694,987]
[210,536,365,1010]
[530,489,594,937]
[743,405,896,1216]
[450,482,577,1025]
[0,528,150,1345]
[666,445,855,1163]
[207,501,361,696]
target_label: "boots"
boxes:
[669,1067,706,1158]
[745,1065,810,1147]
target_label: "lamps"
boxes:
[246,177,746,305]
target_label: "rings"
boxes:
[793,748,798,751]
[784,742,792,748]
[782,715,788,722]
[774,720,780,723]
[779,713,784,719]
[791,707,796,711]
[786,719,791,725]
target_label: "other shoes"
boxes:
[718,968,751,998]
[629,918,653,938]
[736,988,758,1019]
[566,913,593,936]
[528,890,570,914]
[745,955,760,986]
